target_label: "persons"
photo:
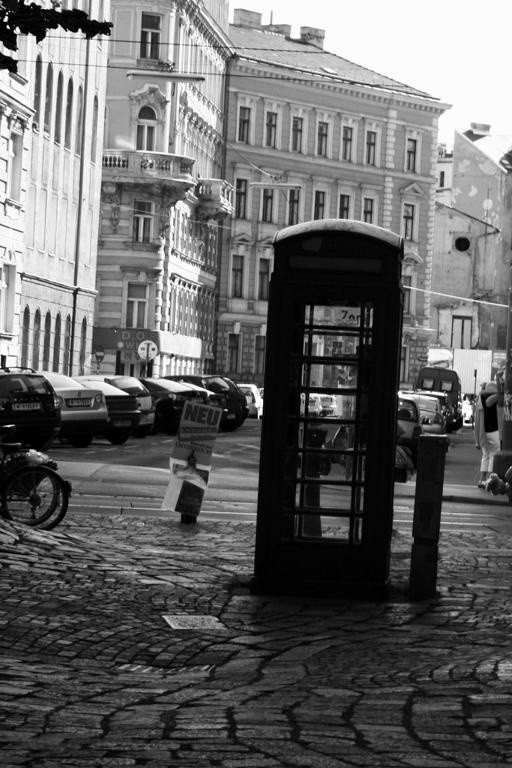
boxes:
[478,382,500,488]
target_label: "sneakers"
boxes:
[478,480,487,488]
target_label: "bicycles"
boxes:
[0,424,71,530]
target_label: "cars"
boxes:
[185,381,228,428]
[140,377,207,430]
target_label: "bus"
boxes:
[416,366,464,428]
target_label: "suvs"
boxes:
[81,372,155,437]
[236,381,359,419]
[397,390,460,456]
[35,369,108,447]
[0,366,62,447]
[166,375,247,433]
[78,380,139,445]
